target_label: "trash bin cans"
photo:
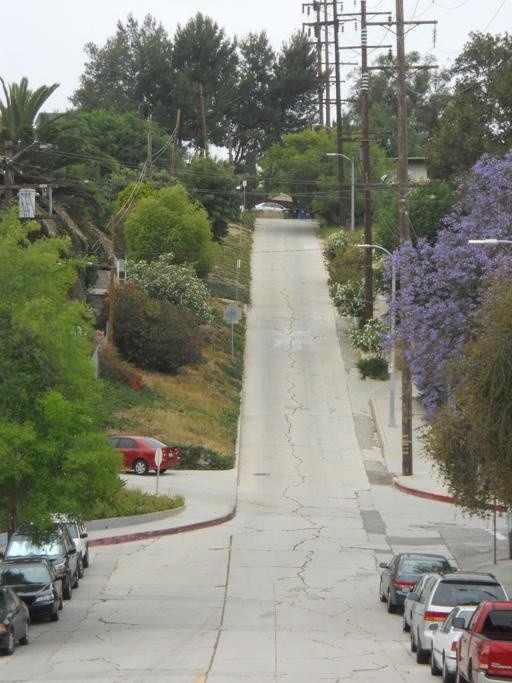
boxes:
[282,209,307,219]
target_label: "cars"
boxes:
[240,200,287,212]
[0,514,89,652]
[107,435,178,475]
[379,552,511,681]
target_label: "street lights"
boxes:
[468,238,508,249]
[328,151,355,233]
[354,242,399,427]
